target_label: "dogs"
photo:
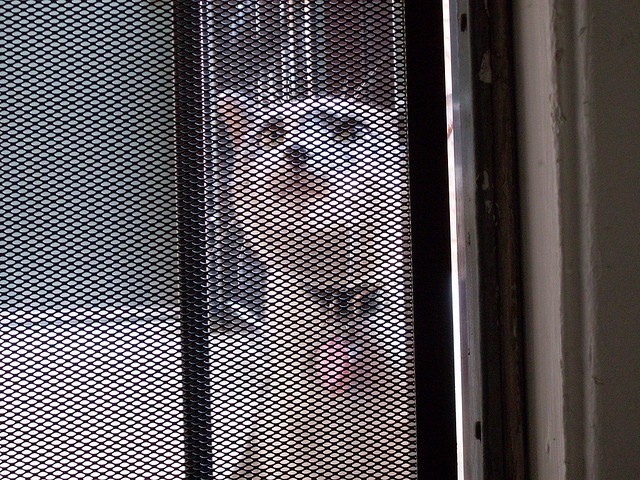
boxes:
[216,82,411,474]
